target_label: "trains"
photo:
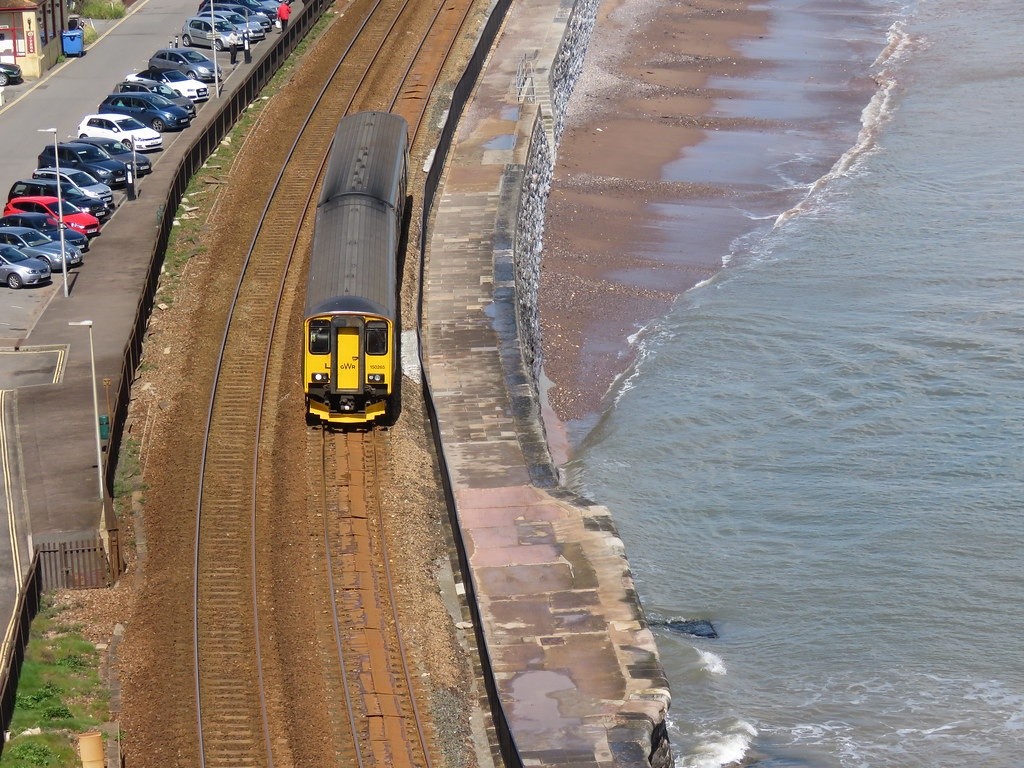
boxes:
[295,109,415,434]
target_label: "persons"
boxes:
[278,2,291,32]
[226,30,238,64]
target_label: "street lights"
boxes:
[67,320,104,503]
[37,127,68,298]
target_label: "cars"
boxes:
[97,91,191,133]
[0,63,22,87]
[77,113,164,152]
[123,67,210,103]
[0,226,83,273]
[70,137,153,178]
[182,15,245,52]
[198,0,294,32]
[198,10,267,44]
[0,211,89,253]
[0,243,52,290]
[113,78,197,121]
[3,195,101,238]
[148,48,223,83]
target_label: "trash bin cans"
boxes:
[62,29,83,57]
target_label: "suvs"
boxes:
[8,177,111,222]
[38,142,130,190]
[32,165,114,206]
